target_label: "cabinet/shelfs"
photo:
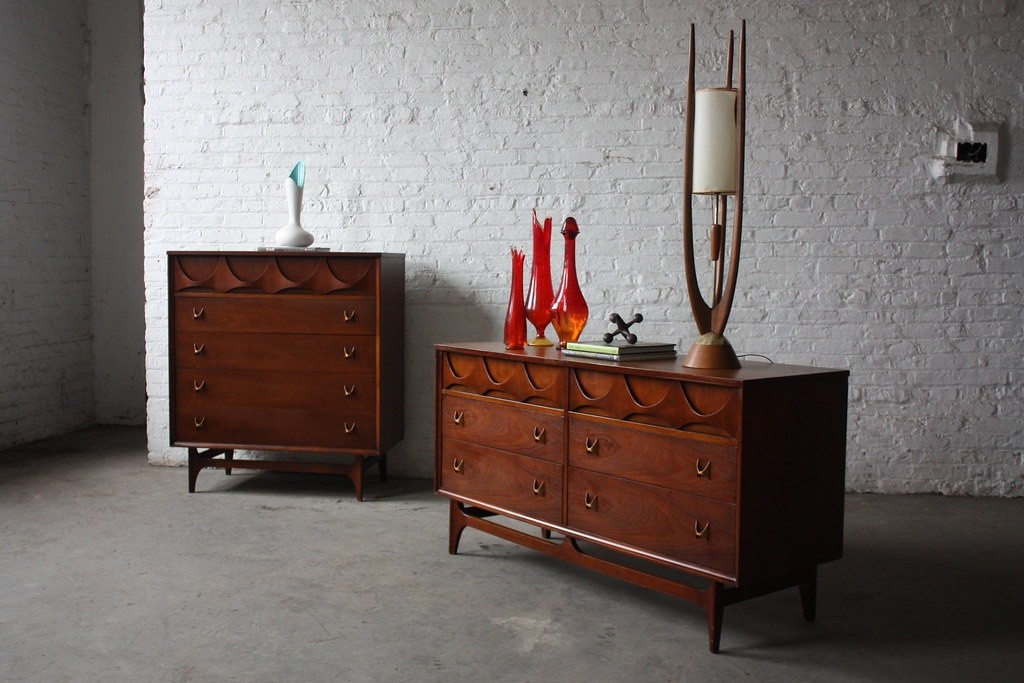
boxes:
[166,251,406,503]
[432,342,849,653]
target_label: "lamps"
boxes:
[682,18,747,368]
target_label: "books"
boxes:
[560,338,677,363]
